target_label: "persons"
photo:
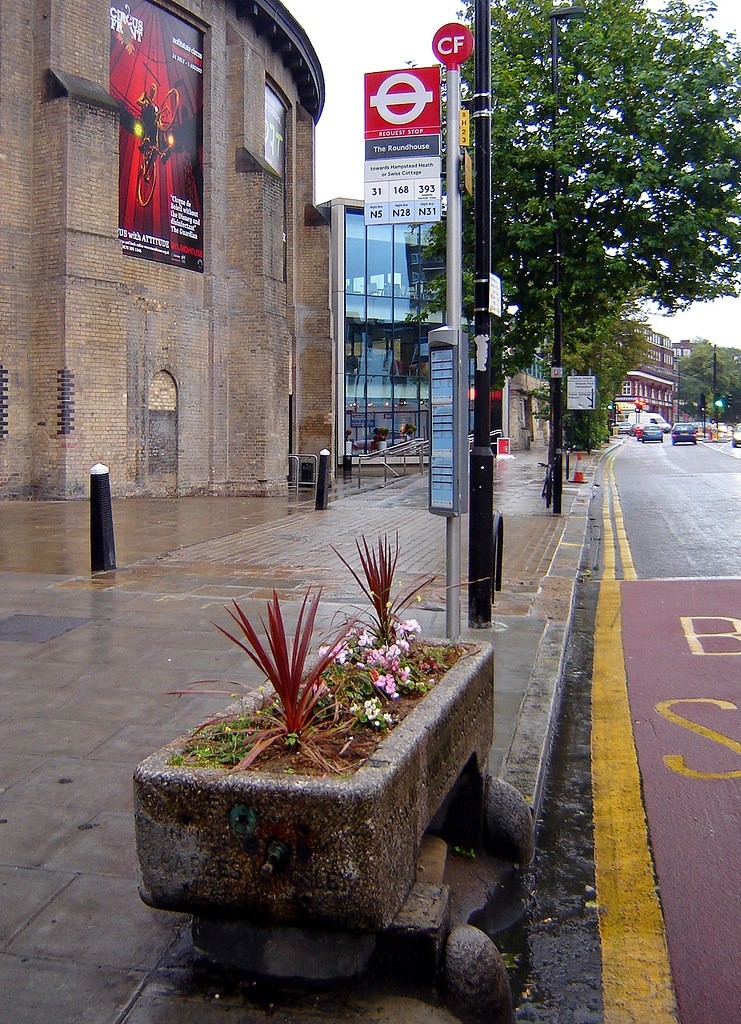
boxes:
[137,83,173,165]
[711,419,717,427]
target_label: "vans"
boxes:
[627,412,670,434]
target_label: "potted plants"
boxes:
[345,428,353,455]
[402,422,417,441]
[377,428,389,451]
[371,428,380,450]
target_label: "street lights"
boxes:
[549,6,585,515]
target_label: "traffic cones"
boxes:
[569,452,587,484]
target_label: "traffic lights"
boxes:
[715,400,723,407]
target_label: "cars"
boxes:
[731,423,741,448]
[672,423,697,445]
[618,422,631,434]
[629,423,663,443]
